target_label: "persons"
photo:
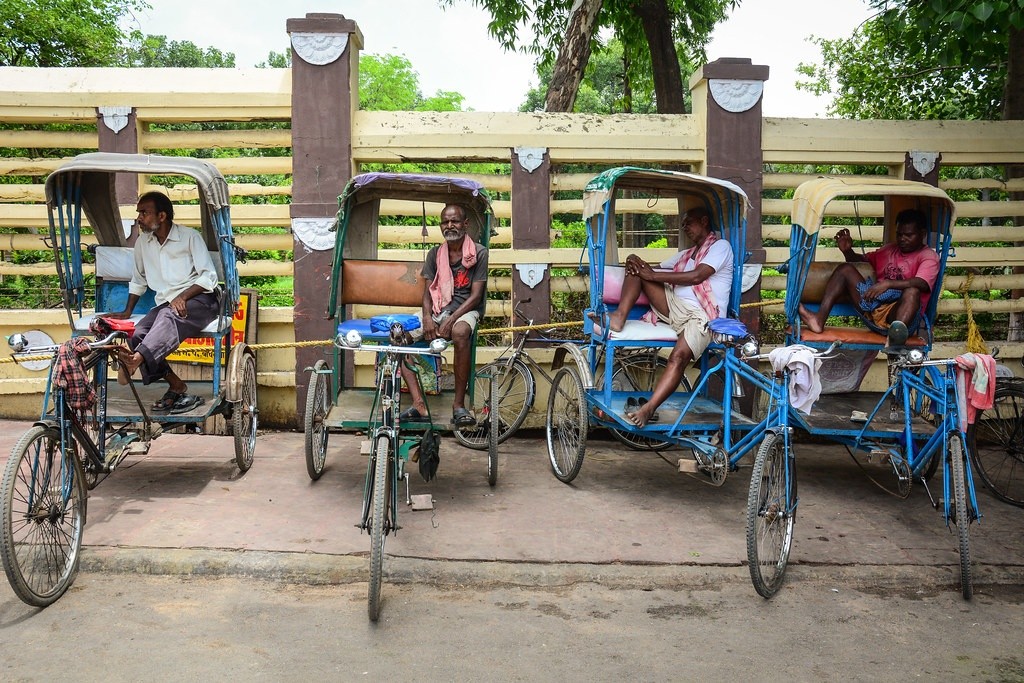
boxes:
[391,204,488,427]
[796,208,941,356]
[95,191,222,412]
[584,206,736,430]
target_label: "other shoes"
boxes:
[638,395,659,421]
[888,320,908,360]
[625,397,641,414]
[849,409,868,422]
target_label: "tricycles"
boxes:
[301,173,499,624]
[552,167,841,599]
[1,151,259,606]
[757,174,999,598]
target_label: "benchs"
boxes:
[791,264,933,354]
[94,246,241,396]
[587,265,682,407]
[337,256,475,396]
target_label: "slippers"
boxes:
[449,408,477,426]
[170,393,205,413]
[391,407,432,423]
[151,390,189,411]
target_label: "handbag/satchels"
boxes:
[374,340,443,395]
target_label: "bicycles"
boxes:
[967,375,1024,508]
[452,298,691,484]
